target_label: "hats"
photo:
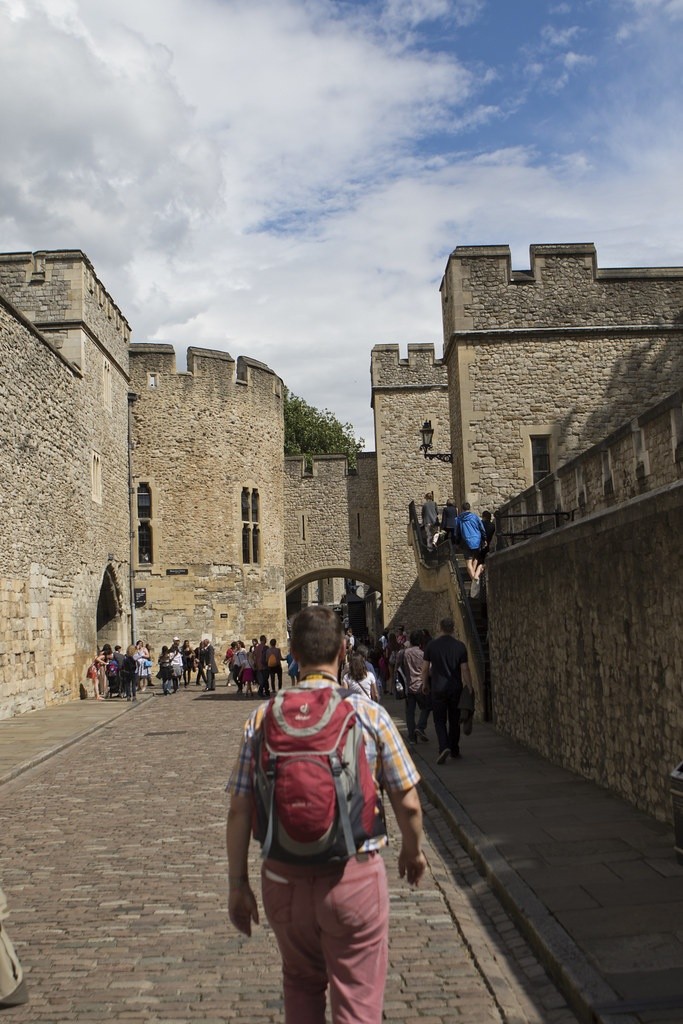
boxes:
[173,637,179,642]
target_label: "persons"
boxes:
[91,618,474,764]
[422,493,494,597]
[225,605,429,1024]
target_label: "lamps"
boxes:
[418,419,455,465]
[351,585,364,589]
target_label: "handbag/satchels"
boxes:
[193,659,197,672]
[0,918,29,1006]
[107,660,118,678]
[379,650,390,681]
[144,661,152,666]
[395,649,406,700]
[471,564,485,599]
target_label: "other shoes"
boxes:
[408,736,417,744]
[94,691,137,701]
[173,690,177,693]
[164,689,172,696]
[237,688,282,698]
[427,542,437,551]
[203,688,215,692]
[437,747,450,764]
[415,727,429,742]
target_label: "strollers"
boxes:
[104,661,127,699]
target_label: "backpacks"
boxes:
[123,654,136,672]
[249,686,388,865]
[268,648,276,667]
